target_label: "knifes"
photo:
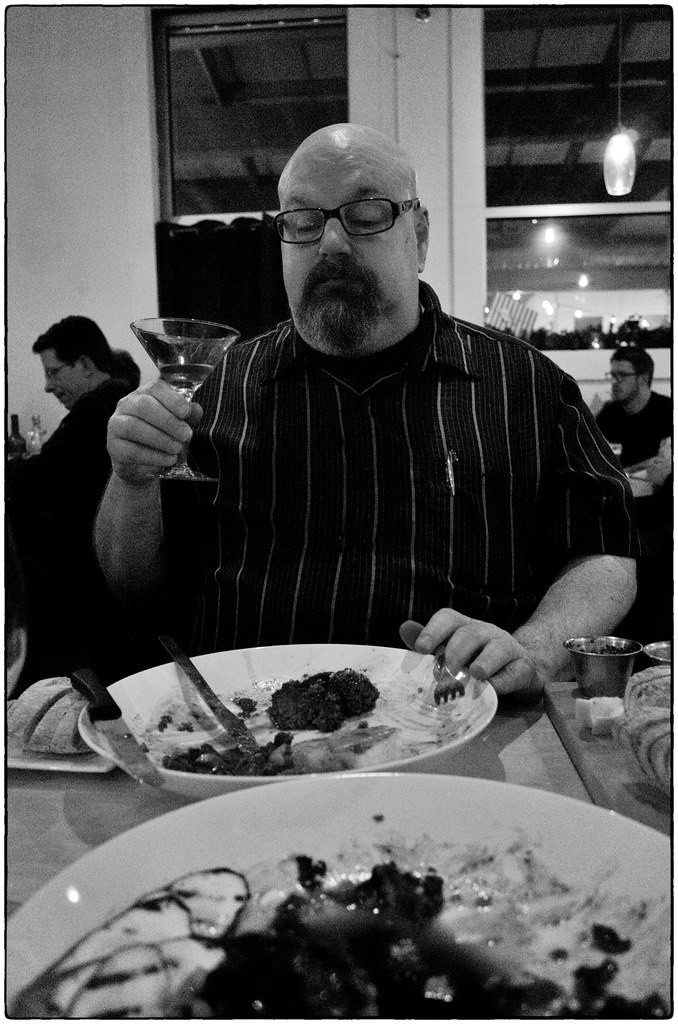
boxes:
[158,632,264,760]
[72,666,166,786]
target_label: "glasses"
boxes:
[606,372,638,382]
[44,364,67,378]
[268,197,421,244]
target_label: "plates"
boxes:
[78,643,499,799]
[8,773,668,1012]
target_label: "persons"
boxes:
[5,313,136,700]
[592,346,673,646]
[95,120,642,701]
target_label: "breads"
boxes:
[610,708,671,799]
[625,665,670,712]
[6,677,91,754]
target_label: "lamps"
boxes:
[603,5,636,197]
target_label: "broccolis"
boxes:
[269,669,378,732]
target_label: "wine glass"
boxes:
[130,319,238,482]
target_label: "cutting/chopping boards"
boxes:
[544,682,671,837]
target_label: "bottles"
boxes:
[26,415,46,454]
[8,414,26,460]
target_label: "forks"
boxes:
[431,641,465,704]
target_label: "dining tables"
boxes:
[5,682,674,1020]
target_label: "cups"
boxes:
[564,637,641,698]
